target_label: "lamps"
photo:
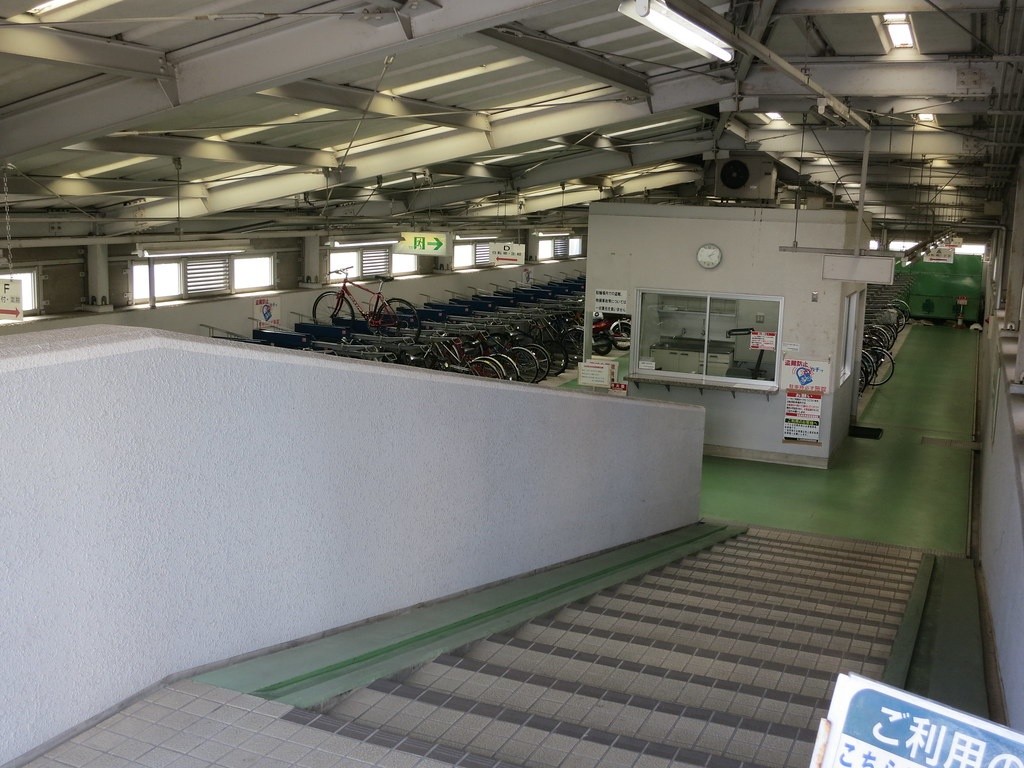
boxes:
[809,104,849,130]
[871,11,921,56]
[323,231,402,249]
[135,239,251,257]
[618,1,748,64]
[532,225,575,238]
[909,113,939,128]
[454,230,503,244]
[751,111,790,126]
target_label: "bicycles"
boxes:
[859,298,910,397]
[312,266,421,345]
[245,267,633,385]
